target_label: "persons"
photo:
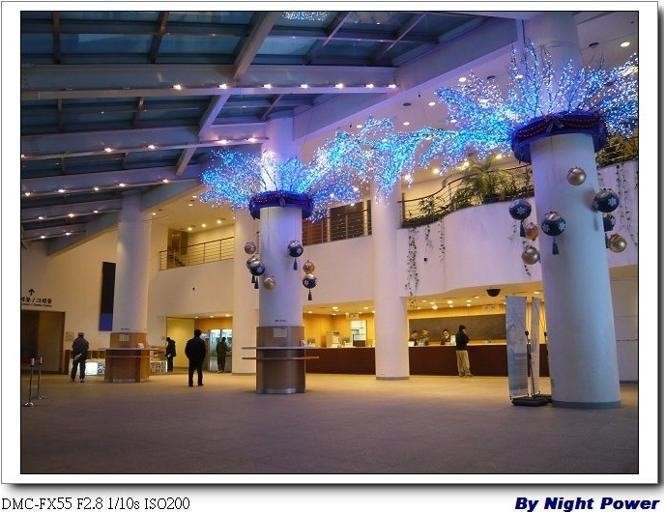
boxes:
[455,324,473,377]
[410,328,431,346]
[440,328,451,345]
[166,337,176,372]
[216,337,230,373]
[185,329,206,387]
[71,332,89,384]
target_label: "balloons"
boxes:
[507,195,566,265]
[263,277,275,289]
[288,238,318,301]
[242,240,265,290]
[568,165,587,187]
[592,184,627,253]
